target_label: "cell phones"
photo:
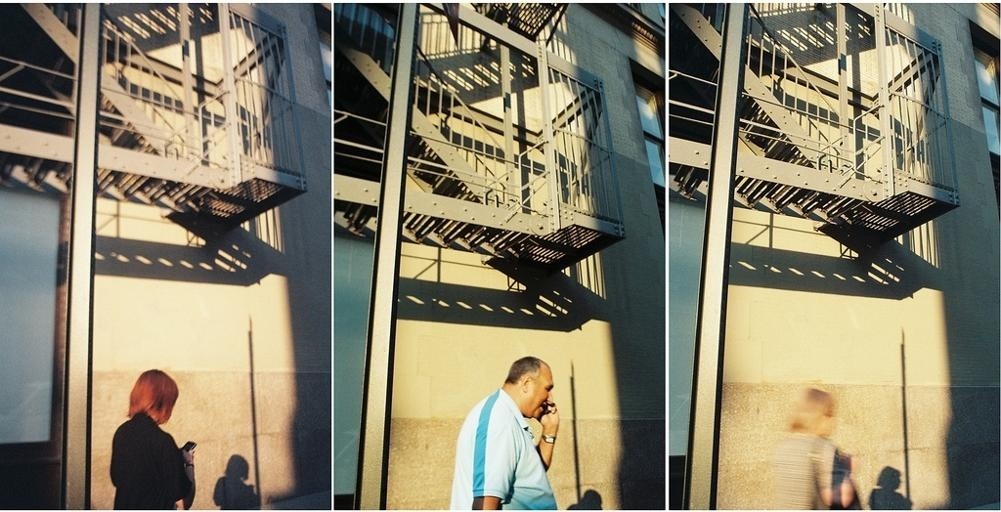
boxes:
[182,441,197,451]
[544,405,552,413]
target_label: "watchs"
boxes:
[541,434,557,444]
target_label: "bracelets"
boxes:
[186,464,194,467]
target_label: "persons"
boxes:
[110,370,195,509]
[450,356,560,509]
[767,388,856,510]
